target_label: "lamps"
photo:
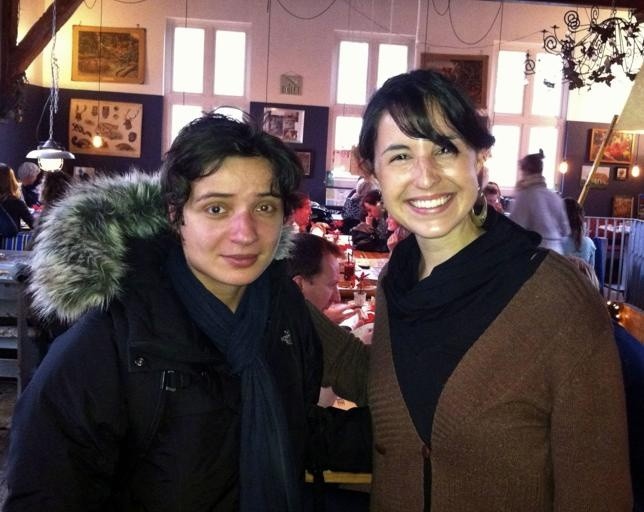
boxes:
[25,0,75,172]
[558,89,570,173]
[632,136,640,177]
[91,0,104,148]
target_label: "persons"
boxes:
[306,67,633,510]
[0,161,77,251]
[0,105,375,512]
[483,148,600,293]
[288,178,410,408]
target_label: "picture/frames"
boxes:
[614,167,628,182]
[70,25,147,84]
[589,129,634,165]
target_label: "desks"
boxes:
[1,206,45,398]
[300,233,391,342]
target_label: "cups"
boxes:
[343,262,356,281]
